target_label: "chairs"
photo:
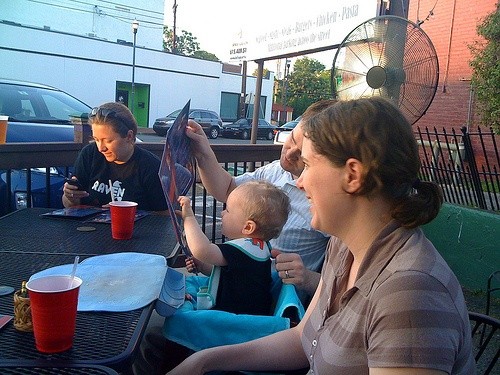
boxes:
[419,202,500,375]
[163,266,306,352]
[0,87,22,116]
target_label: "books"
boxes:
[158,99,196,257]
[39,204,152,224]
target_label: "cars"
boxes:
[274,116,302,145]
[0,76,146,210]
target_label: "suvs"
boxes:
[153,108,224,140]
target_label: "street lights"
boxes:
[279,58,291,107]
[131,17,140,114]
[283,64,291,125]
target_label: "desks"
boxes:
[0,252,166,375]
[0,207,183,264]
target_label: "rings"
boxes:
[72,194,74,198]
[285,270,289,278]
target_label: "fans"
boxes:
[331,14,440,126]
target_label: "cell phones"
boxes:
[67,179,83,191]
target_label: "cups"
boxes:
[25,275,83,353]
[109,201,138,240]
[0,116,9,144]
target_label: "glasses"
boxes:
[92,108,130,130]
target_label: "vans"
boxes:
[221,118,278,140]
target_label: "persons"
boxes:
[166,96,476,374]
[174,182,293,316]
[144,100,339,374]
[63,102,168,211]
